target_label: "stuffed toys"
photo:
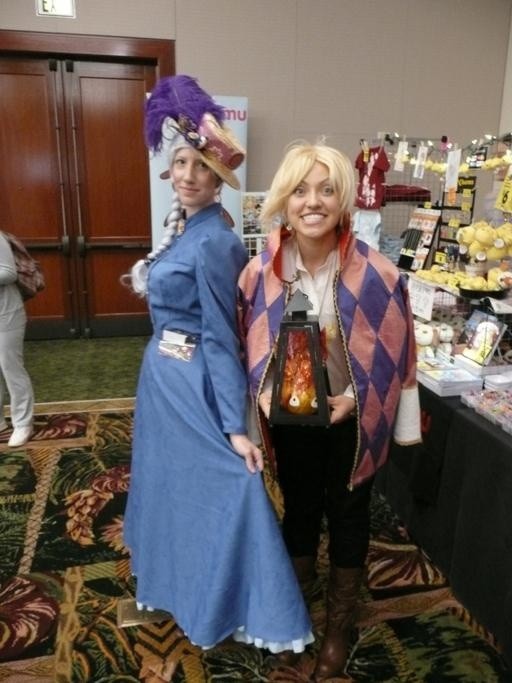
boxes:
[413,320,454,364]
[416,221,512,291]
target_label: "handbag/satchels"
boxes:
[4,230,45,299]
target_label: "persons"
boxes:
[0,235,35,448]
[236,134,424,682]
[121,131,317,654]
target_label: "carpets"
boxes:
[0,397,511,681]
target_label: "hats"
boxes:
[173,111,247,191]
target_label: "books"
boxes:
[455,309,498,351]
[462,318,508,366]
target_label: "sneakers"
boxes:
[7,426,34,448]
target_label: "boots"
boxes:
[313,565,363,682]
[278,555,318,666]
